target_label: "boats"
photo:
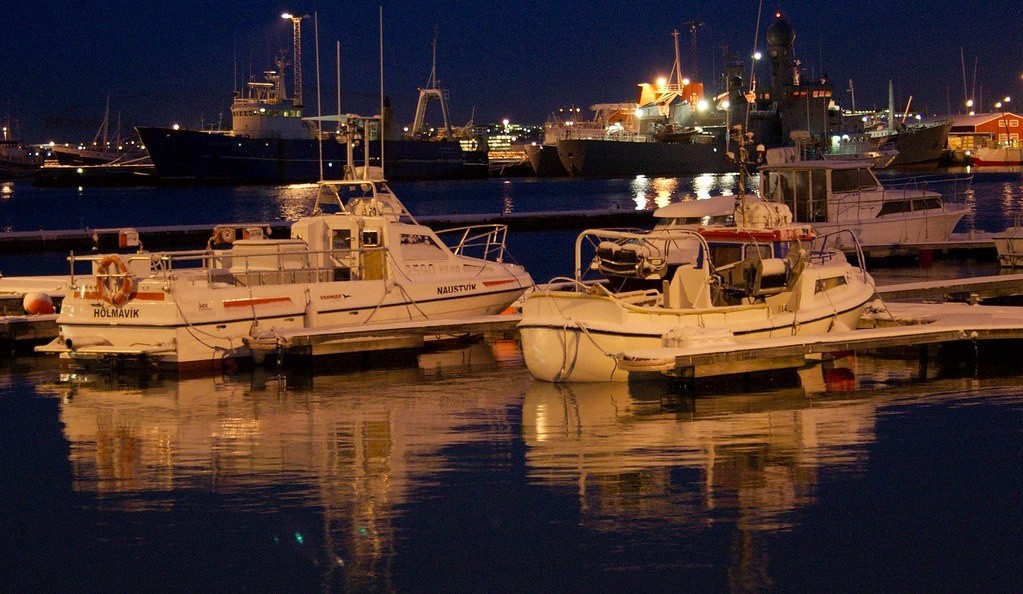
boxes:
[0,139,40,176]
[523,82,957,178]
[766,134,899,168]
[520,359,879,594]
[34,120,533,372]
[969,142,1023,166]
[46,356,526,578]
[756,135,974,250]
[135,82,488,182]
[516,124,874,382]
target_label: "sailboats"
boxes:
[51,95,140,166]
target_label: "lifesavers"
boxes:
[96,255,131,305]
